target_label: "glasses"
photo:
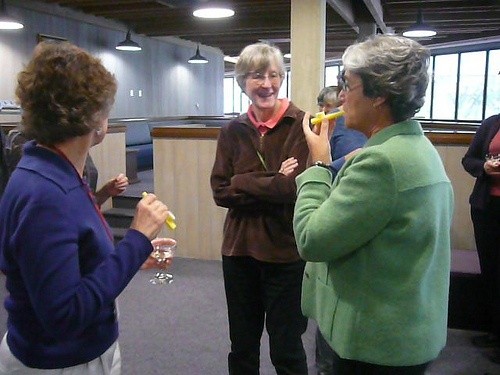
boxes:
[338,76,365,91]
[245,71,282,85]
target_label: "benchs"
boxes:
[117,121,196,170]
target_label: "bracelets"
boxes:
[311,161,338,174]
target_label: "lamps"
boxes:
[0,0,24,30]
[114,27,143,51]
[402,12,437,38]
[192,0,235,19]
[188,42,209,64]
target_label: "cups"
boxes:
[485,152,500,169]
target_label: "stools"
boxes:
[445,249,481,329]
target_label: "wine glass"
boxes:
[150,238,177,286]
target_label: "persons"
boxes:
[0,38,170,374]
[460,112,500,364]
[206,37,457,375]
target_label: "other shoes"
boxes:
[473,334,498,348]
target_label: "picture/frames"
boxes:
[38,33,69,44]
